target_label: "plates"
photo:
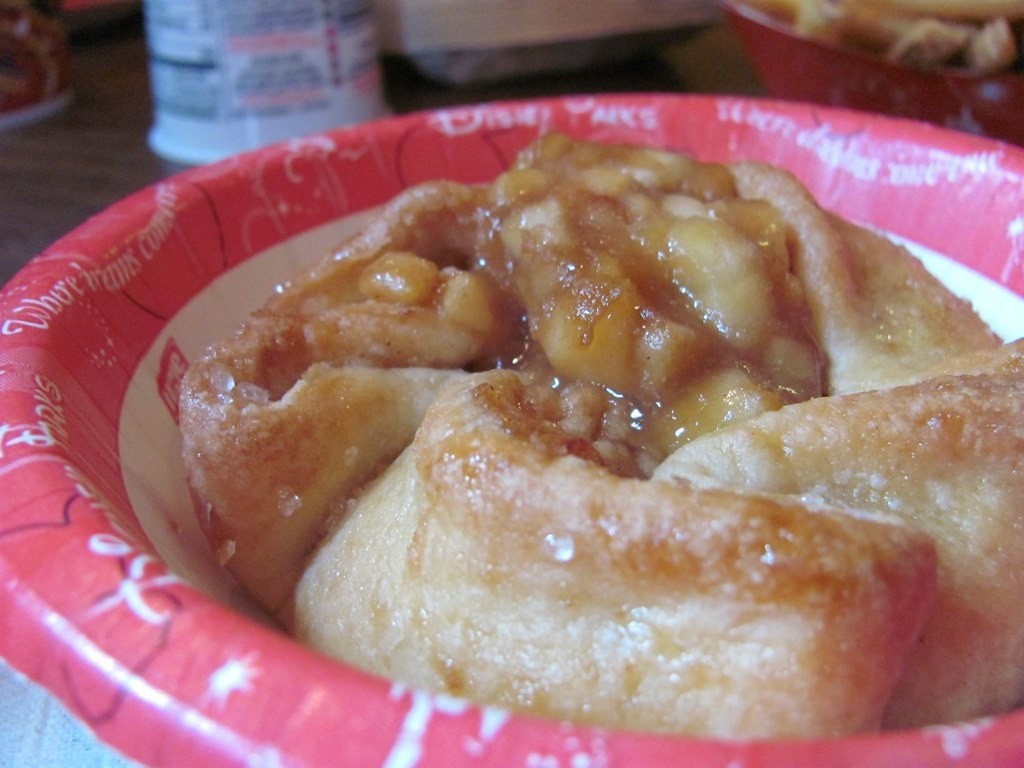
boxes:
[0,0,84,136]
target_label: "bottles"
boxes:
[140,0,397,165]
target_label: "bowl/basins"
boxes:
[372,0,713,87]
[0,93,1024,767]
[718,0,1024,148]
